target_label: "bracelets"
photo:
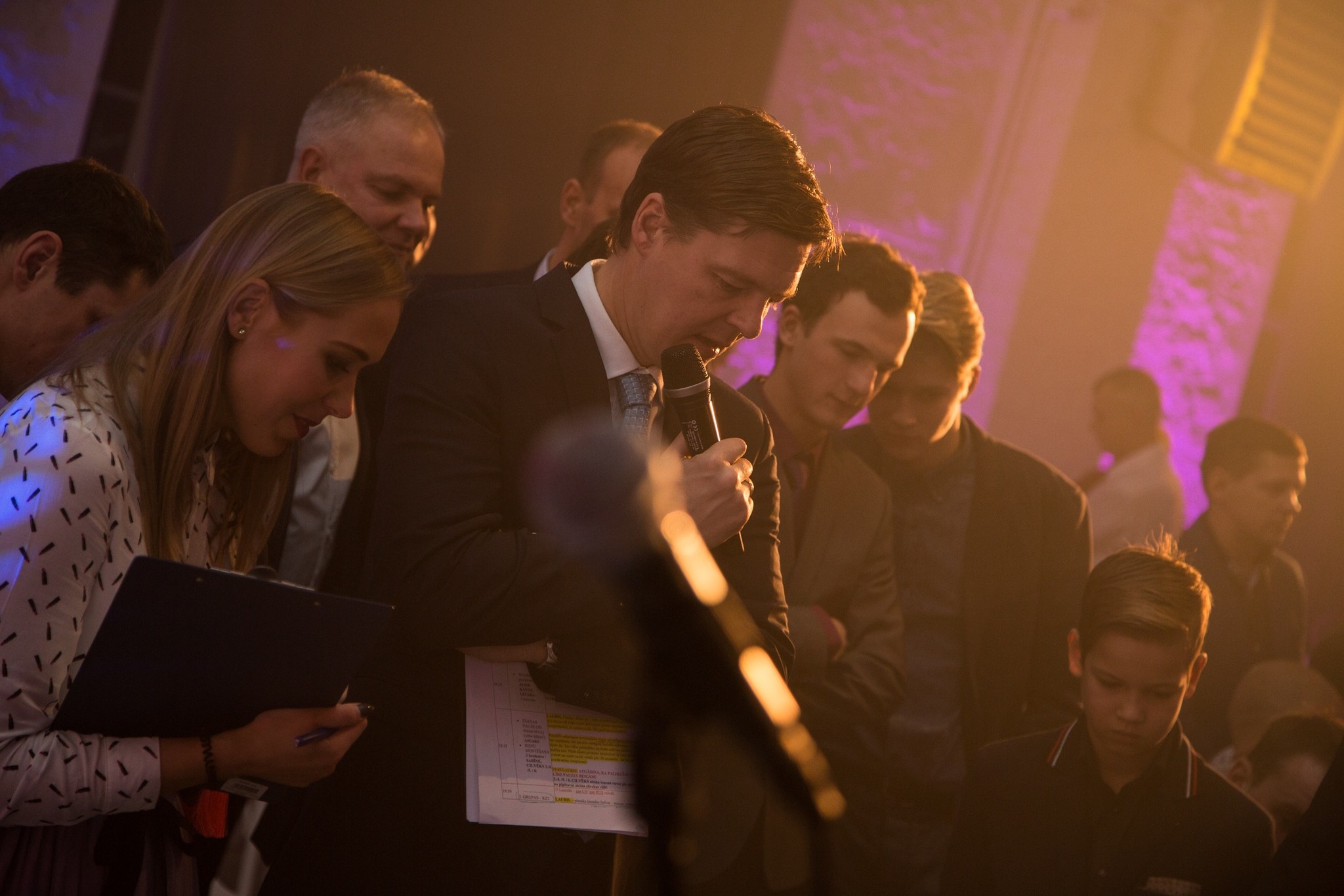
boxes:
[200,731,216,792]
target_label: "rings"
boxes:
[744,478,753,496]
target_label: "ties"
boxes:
[616,373,657,449]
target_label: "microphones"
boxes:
[524,412,850,829]
[659,343,747,556]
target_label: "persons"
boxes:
[239,68,443,589]
[1075,370,1183,566]
[251,107,835,895]
[396,121,670,309]
[1,159,162,410]
[610,236,925,893]
[941,527,1273,896]
[837,269,1090,894]
[2,184,406,896]
[1228,660,1343,849]
[1175,417,1306,764]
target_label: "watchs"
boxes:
[533,636,563,672]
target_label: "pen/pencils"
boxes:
[294,726,343,748]
